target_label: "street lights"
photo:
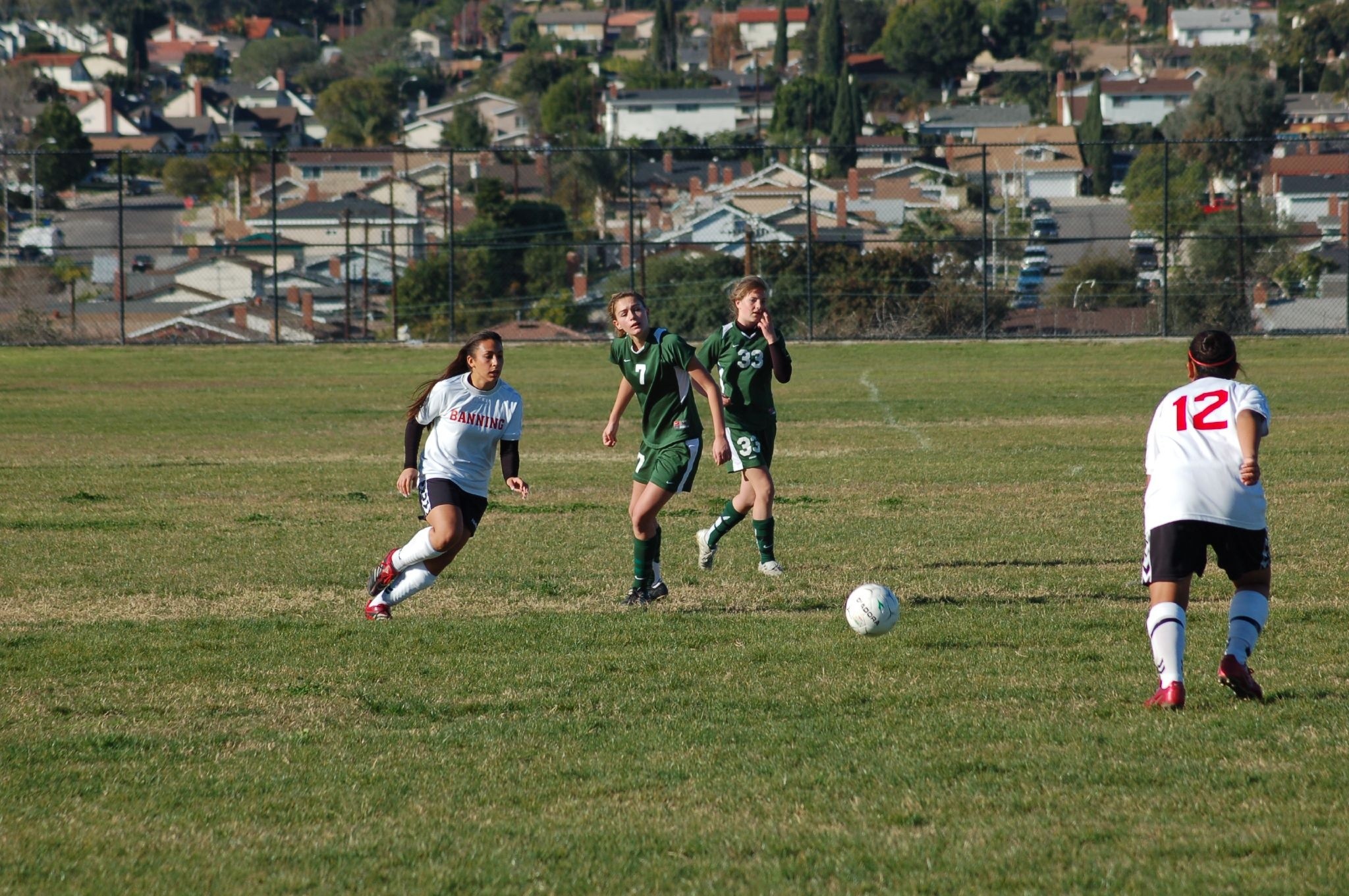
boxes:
[30,138,57,226]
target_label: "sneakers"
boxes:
[648,579,669,600]
[368,548,401,596]
[696,529,718,571]
[1145,680,1186,707]
[621,588,649,607]
[758,560,784,576]
[365,600,396,621]
[1217,656,1263,701]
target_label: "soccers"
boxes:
[845,582,901,637]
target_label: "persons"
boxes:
[362,330,529,620]
[600,290,733,608]
[686,275,792,576]
[1137,328,1272,711]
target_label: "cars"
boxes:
[1127,228,1165,304]
[1011,198,1060,314]
[124,179,151,195]
[131,255,156,269]
